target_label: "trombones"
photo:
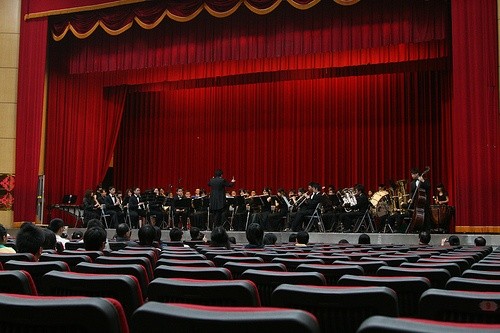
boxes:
[291,190,311,210]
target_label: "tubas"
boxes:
[337,188,359,213]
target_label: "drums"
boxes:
[430,205,455,232]
[370,191,389,216]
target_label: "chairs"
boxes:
[125,203,132,229]
[99,204,110,228]
[0,241,500,333]
[304,203,412,233]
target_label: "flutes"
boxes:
[95,198,105,215]
[114,193,124,211]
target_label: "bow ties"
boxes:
[112,196,115,197]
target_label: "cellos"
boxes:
[405,167,430,230]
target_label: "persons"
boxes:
[418,231,431,245]
[47,218,70,249]
[339,233,371,244]
[264,233,277,245]
[81,168,449,232]
[190,227,200,240]
[441,235,460,246]
[16,224,45,262]
[72,230,83,242]
[87,219,105,230]
[169,228,189,247]
[244,223,265,248]
[0,224,16,254]
[41,228,56,255]
[83,227,106,251]
[289,230,309,247]
[210,227,231,247]
[474,236,486,246]
[113,223,162,251]
